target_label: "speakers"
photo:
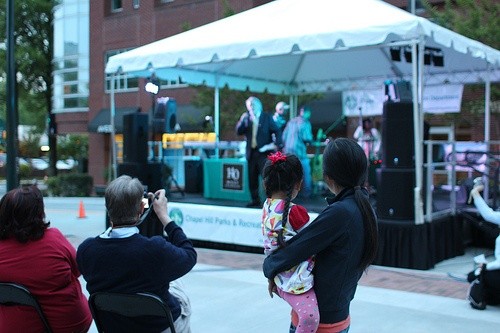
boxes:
[122,112,149,164]
[116,162,162,201]
[376,168,427,221]
[381,100,415,168]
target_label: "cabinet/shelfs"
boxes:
[423,140,457,221]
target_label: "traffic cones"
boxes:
[79,201,85,218]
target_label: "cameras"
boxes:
[140,184,150,208]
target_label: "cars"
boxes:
[0,153,79,179]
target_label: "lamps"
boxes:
[390,40,445,67]
[145,70,161,94]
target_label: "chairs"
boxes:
[0,282,53,333]
[88,293,176,333]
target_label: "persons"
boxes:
[262,137,379,333]
[262,151,320,333]
[76,174,197,333]
[0,184,93,333]
[352,116,382,197]
[272,102,291,145]
[282,105,314,199]
[235,96,285,208]
[466,183,500,311]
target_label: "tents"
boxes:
[104,0,500,224]
